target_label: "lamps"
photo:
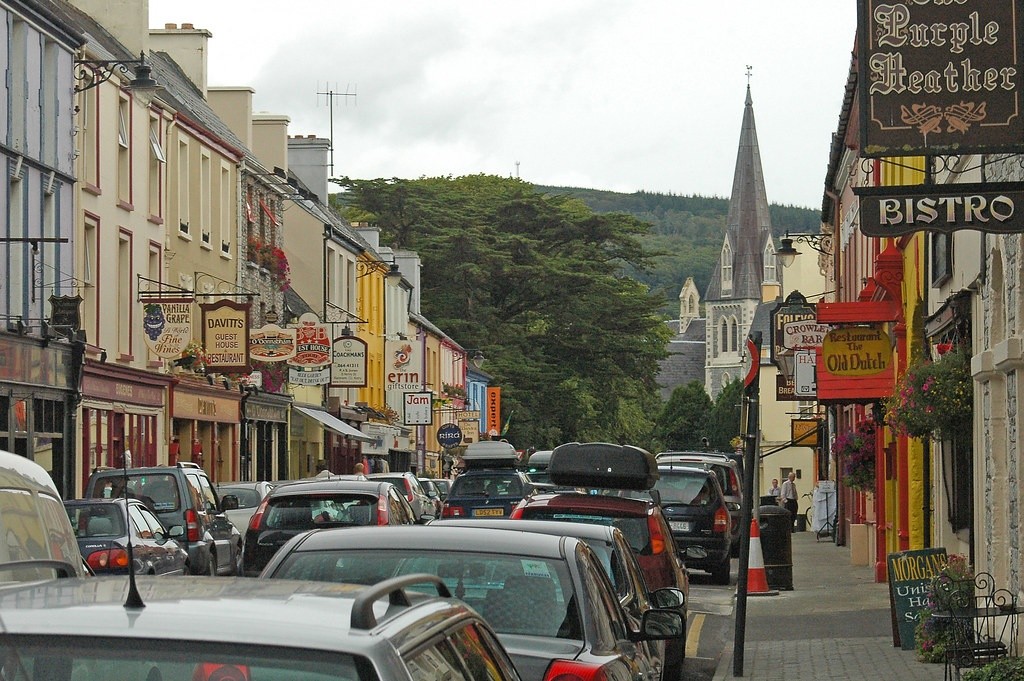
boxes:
[0,313,108,366]
[74,50,165,110]
[248,166,318,212]
[174,356,196,367]
[248,384,259,396]
[771,231,836,281]
[220,373,231,390]
[453,345,484,368]
[356,256,402,287]
[776,349,796,381]
[197,371,213,387]
[340,405,386,419]
[31,239,40,256]
[457,399,471,409]
[239,383,245,394]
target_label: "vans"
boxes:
[0,450,97,580]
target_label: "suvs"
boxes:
[214,440,744,596]
[85,461,243,576]
[0,558,520,681]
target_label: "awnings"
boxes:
[260,201,279,226]
[294,405,376,444]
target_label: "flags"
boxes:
[501,412,513,436]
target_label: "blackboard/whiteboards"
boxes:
[888,546,952,649]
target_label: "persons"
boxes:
[316,465,334,477]
[394,436,398,448]
[769,478,782,505]
[355,463,364,474]
[781,472,799,533]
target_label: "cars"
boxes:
[188,515,687,681]
[63,499,192,577]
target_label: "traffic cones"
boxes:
[734,518,779,597]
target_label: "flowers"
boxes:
[829,412,878,502]
[879,341,973,443]
[915,553,974,664]
[440,380,467,398]
[182,340,290,394]
[246,233,292,293]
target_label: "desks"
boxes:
[931,607,1024,617]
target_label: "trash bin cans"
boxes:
[752,504,794,592]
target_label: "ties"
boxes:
[791,483,795,498]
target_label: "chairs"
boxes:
[684,482,708,495]
[87,518,113,535]
[482,576,557,629]
[935,573,1019,681]
